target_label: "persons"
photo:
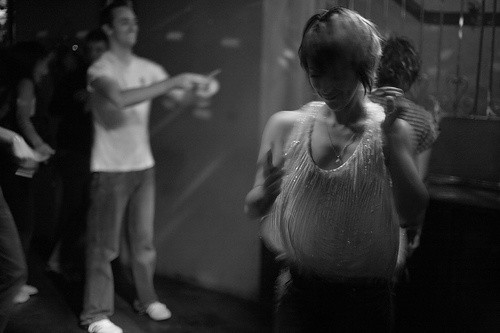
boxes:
[0,30,124,304]
[244,7,432,332]
[365,36,439,272]
[84,4,218,333]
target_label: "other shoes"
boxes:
[88,316,124,332]
[12,283,39,302]
[134,300,171,320]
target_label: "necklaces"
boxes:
[321,117,358,164]
